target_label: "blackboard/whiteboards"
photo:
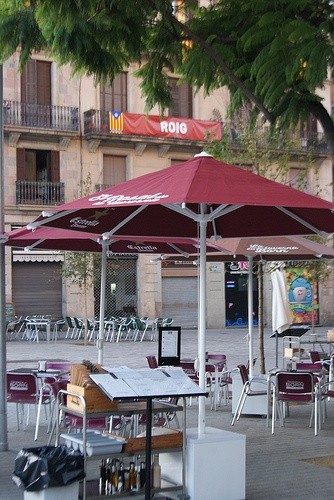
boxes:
[3,303,15,321]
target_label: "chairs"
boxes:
[309,380,334,428]
[287,362,322,417]
[232,364,282,428]
[4,355,179,446]
[218,357,258,407]
[208,354,230,403]
[308,351,330,370]
[269,370,318,437]
[6,314,175,344]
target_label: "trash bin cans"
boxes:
[22,446,80,500]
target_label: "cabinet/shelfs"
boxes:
[58,404,187,500]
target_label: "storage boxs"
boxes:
[65,383,118,414]
[105,426,183,453]
[86,459,102,480]
[79,480,99,496]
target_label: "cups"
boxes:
[309,334,317,342]
[206,352,208,362]
[38,361,48,372]
[327,333,334,340]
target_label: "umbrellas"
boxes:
[270,259,292,367]
[27,151,334,438]
[153,236,334,378]
[0,226,231,365]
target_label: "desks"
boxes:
[265,368,327,428]
[180,357,227,412]
[7,368,70,431]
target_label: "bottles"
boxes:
[98,456,146,495]
[152,454,161,488]
